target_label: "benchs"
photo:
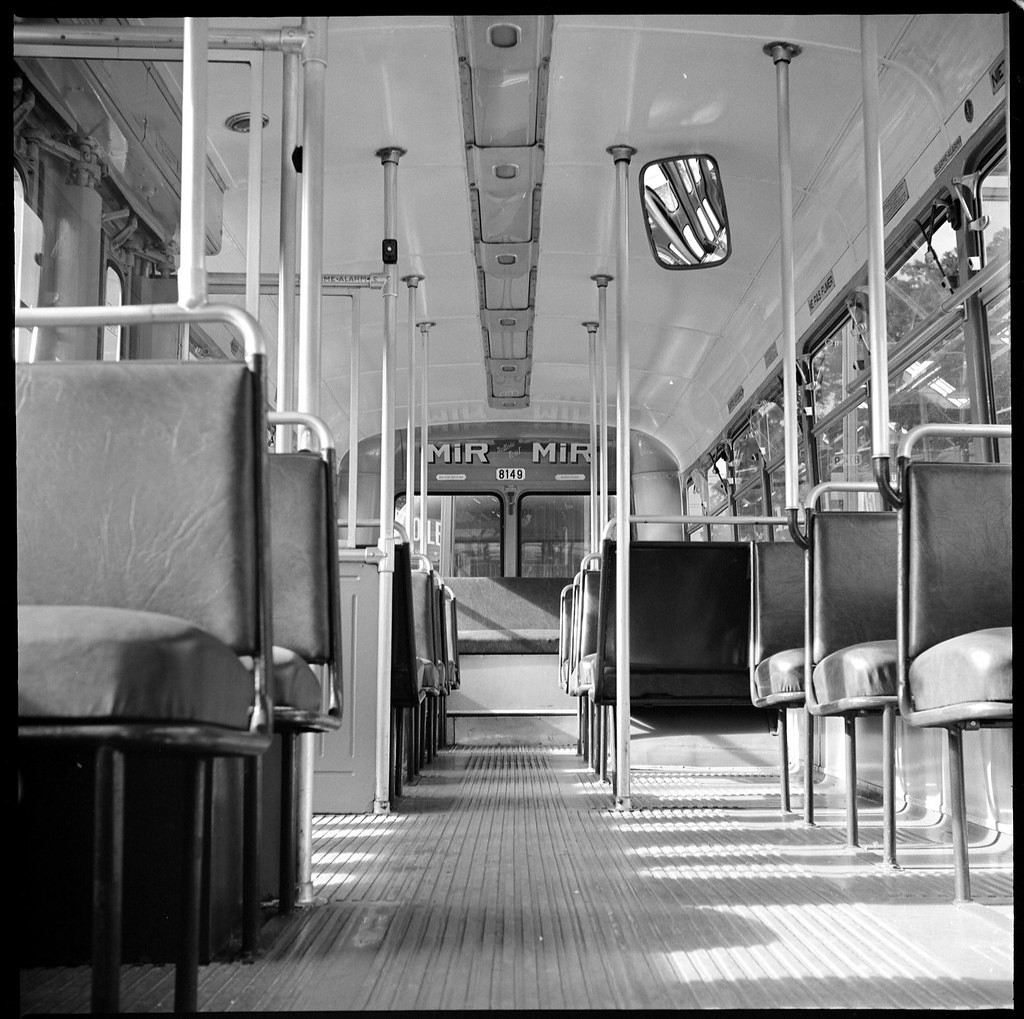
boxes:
[336,518,460,811]
[441,576,573,654]
[16,304,271,1014]
[17,411,337,966]
[559,515,805,800]
[883,424,1014,907]
[800,483,898,847]
